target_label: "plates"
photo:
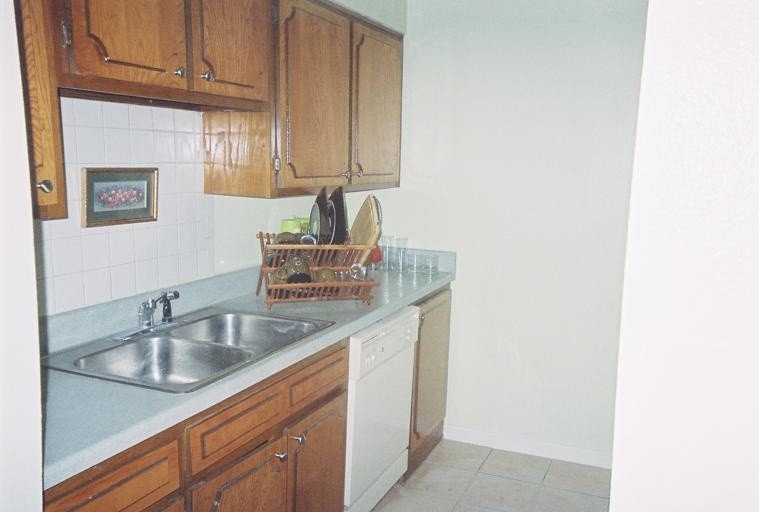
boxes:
[309,187,348,245]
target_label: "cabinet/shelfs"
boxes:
[43,286,453,512]
[13,1,404,220]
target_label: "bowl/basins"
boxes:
[273,233,299,244]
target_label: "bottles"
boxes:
[334,269,361,282]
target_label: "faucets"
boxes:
[138,290,181,330]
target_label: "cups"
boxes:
[380,236,438,274]
[275,257,312,283]
[315,268,337,285]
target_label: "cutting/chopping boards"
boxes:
[349,193,382,266]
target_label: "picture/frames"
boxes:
[80,165,159,229]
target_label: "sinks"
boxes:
[40,330,254,394]
[169,306,337,351]
